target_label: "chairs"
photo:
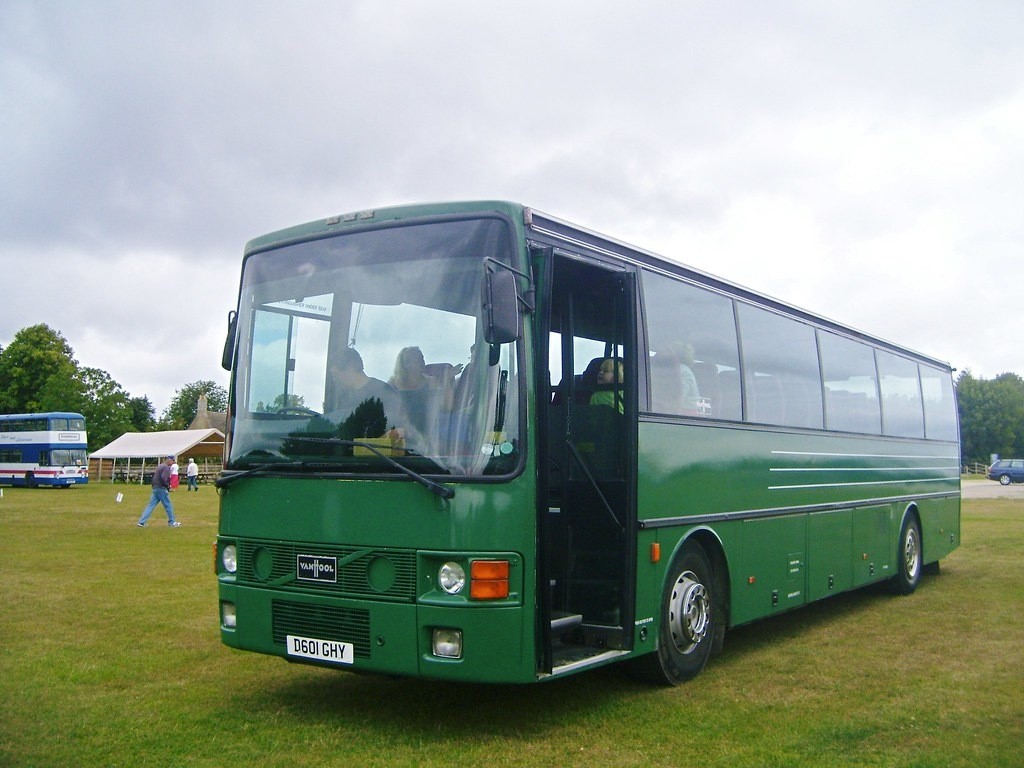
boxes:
[554,355,957,441]
[423,360,455,414]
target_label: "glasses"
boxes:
[468,357,476,360]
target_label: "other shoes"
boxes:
[195,488,198,492]
[188,487,192,492]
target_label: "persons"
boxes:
[187,458,198,491]
[170,461,179,490]
[576,359,624,451]
[655,341,701,409]
[387,346,464,413]
[458,345,500,414]
[328,349,426,444]
[138,455,182,527]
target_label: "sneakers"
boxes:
[169,522,182,527]
[138,523,149,528]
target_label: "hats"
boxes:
[167,455,176,460]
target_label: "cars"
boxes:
[986,459,1024,485]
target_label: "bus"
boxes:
[0,412,89,489]
[213,200,962,687]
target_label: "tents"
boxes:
[88,428,224,486]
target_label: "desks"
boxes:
[112,470,213,483]
